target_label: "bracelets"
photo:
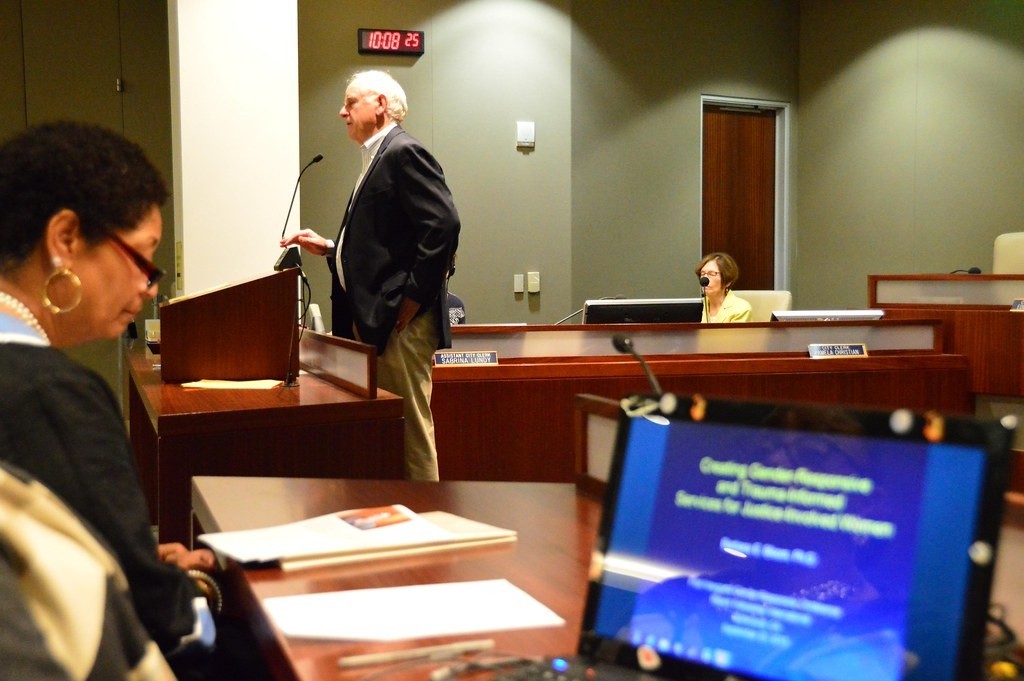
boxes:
[186,567,223,616]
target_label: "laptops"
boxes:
[483,392,1014,681]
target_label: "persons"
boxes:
[696,252,752,323]
[0,118,223,680]
[281,70,460,480]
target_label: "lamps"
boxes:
[516,120,536,149]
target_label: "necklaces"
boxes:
[0,288,54,348]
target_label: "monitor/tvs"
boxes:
[770,309,886,320]
[582,298,705,323]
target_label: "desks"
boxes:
[867,273,1023,391]
[116,320,407,558]
[406,319,973,486]
[189,474,611,681]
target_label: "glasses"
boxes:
[82,211,166,288]
[701,271,721,277]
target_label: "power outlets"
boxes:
[513,274,524,292]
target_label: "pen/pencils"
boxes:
[337,638,495,667]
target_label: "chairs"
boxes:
[727,290,791,320]
[993,233,1023,304]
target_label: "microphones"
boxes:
[274,153,323,272]
[700,277,710,322]
[612,333,664,397]
